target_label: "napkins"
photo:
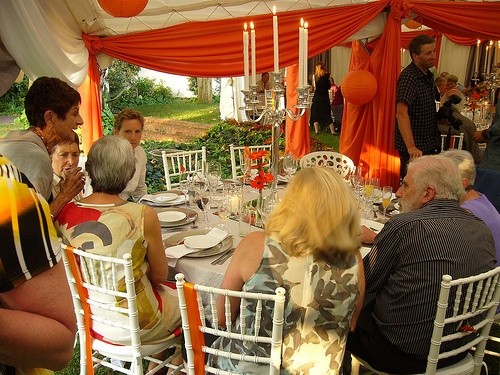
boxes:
[164,226,229,268]
[135,193,188,205]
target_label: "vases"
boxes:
[471,111,476,122]
[480,103,487,120]
[255,189,265,225]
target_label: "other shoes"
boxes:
[331,132,337,136]
[315,132,319,136]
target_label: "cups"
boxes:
[345,167,380,220]
[188,183,200,209]
[227,182,285,237]
[204,162,221,180]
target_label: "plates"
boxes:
[184,235,218,250]
[375,190,400,216]
[153,192,178,201]
[157,210,186,222]
[143,190,189,206]
[151,207,198,228]
[165,225,234,258]
[239,177,252,184]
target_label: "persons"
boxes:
[0,36,500,375]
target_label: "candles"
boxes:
[231,194,240,212]
[298,17,305,89]
[303,22,309,87]
[486,40,493,74]
[249,21,256,85]
[242,22,249,91]
[475,39,480,72]
[272,4,279,73]
[494,40,500,66]
[484,46,489,69]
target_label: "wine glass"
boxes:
[179,179,191,204]
[382,185,393,218]
[240,159,249,186]
[200,175,232,229]
[283,154,299,177]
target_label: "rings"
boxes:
[81,178,85,182]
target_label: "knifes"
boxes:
[212,248,236,265]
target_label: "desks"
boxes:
[138,173,401,334]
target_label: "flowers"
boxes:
[238,128,287,219]
[464,89,491,123]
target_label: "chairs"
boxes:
[351,266,500,375]
[174,273,286,375]
[161,146,207,190]
[228,143,273,179]
[58,237,187,375]
[440,132,464,154]
[299,151,356,182]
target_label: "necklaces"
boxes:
[29,126,51,156]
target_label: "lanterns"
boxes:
[403,20,421,29]
[340,69,377,105]
[97,0,149,18]
